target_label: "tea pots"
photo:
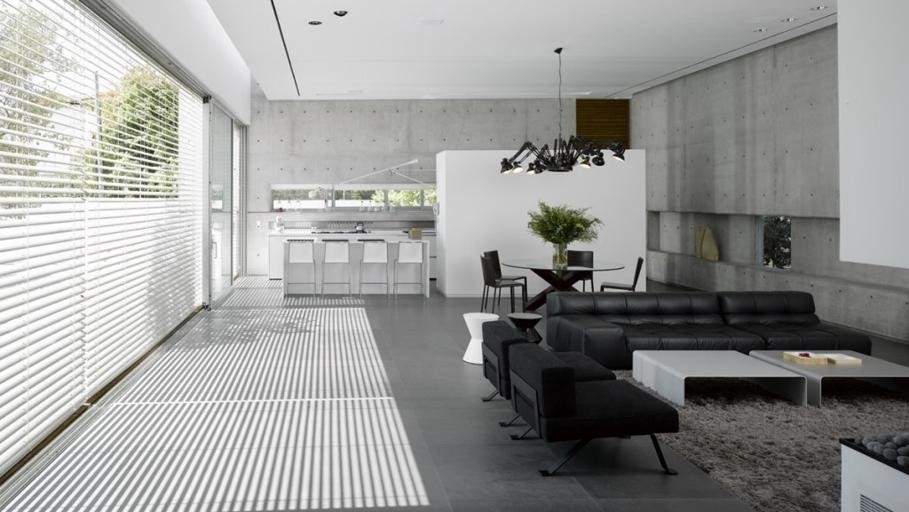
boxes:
[354,222,366,233]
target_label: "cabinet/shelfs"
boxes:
[421,234,437,279]
[269,234,283,281]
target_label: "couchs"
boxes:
[549,289,872,370]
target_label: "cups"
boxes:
[311,226,316,233]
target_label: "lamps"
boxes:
[308,158,439,214]
[498,46,625,174]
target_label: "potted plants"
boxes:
[521,197,606,266]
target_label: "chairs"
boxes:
[321,241,352,299]
[393,242,424,301]
[506,343,683,479]
[600,256,645,292]
[358,241,389,302]
[476,319,615,427]
[285,239,317,298]
[567,249,594,292]
[484,250,528,305]
[479,254,526,315]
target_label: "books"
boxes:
[818,353,862,366]
[785,350,828,364]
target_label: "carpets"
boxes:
[609,367,909,512]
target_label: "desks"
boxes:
[501,256,626,312]
[506,311,545,345]
[461,312,501,366]
[283,237,431,298]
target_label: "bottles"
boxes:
[273,216,284,233]
[326,221,374,230]
[358,199,395,213]
[274,197,303,213]
